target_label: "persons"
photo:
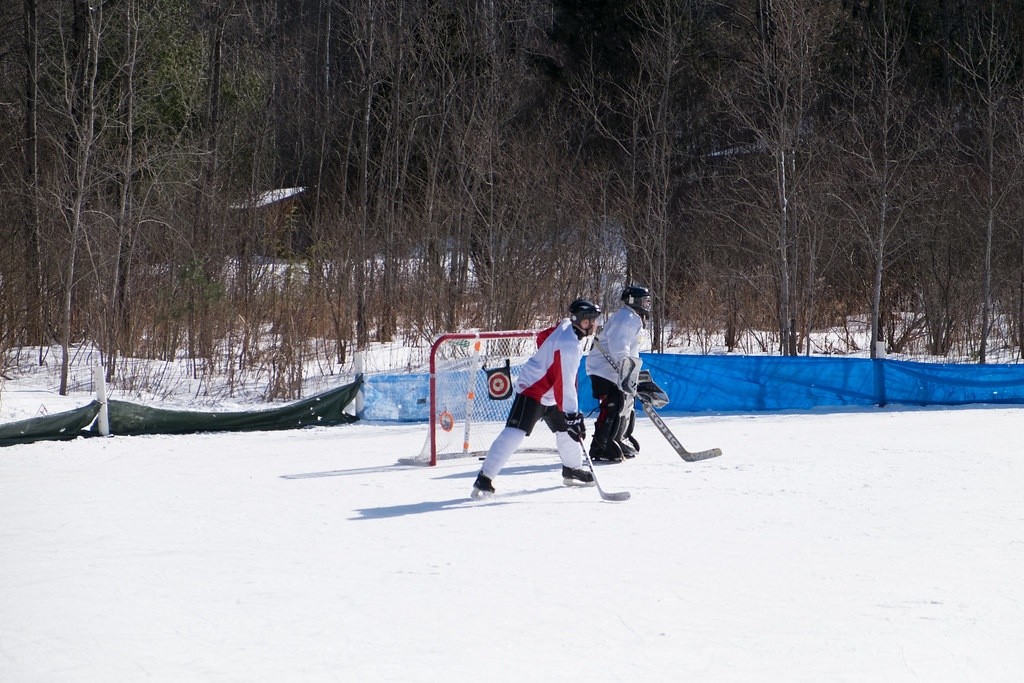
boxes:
[470,299,604,502]
[587,286,670,462]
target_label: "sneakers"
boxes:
[562,466,595,487]
[470,472,495,499]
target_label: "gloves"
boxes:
[564,413,586,442]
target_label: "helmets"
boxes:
[622,286,651,317]
[569,301,602,324]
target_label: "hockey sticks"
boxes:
[579,439,631,501]
[592,337,723,464]
